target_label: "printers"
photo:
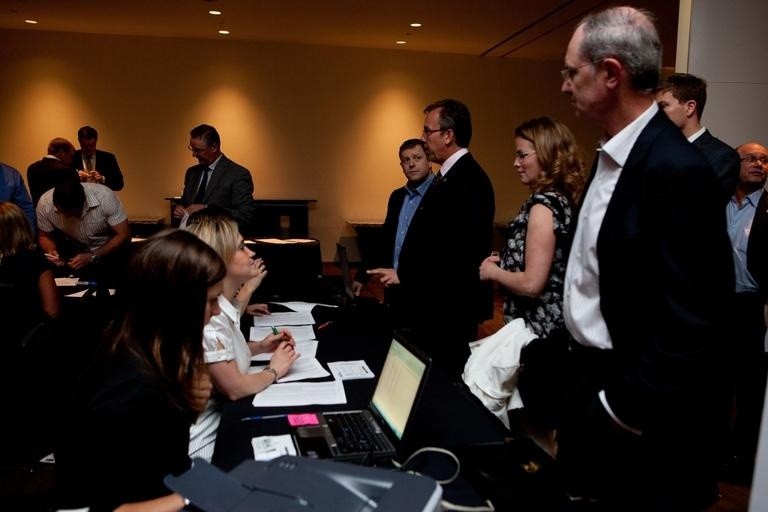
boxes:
[161,456,444,512]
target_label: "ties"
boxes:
[429,170,442,190]
[193,168,210,204]
[84,154,94,174]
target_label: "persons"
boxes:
[350,138,437,316]
[369,100,497,372]
[2,201,59,363]
[174,124,256,230]
[33,178,139,323]
[653,71,743,201]
[64,126,124,191]
[27,138,75,209]
[478,116,588,452]
[231,258,271,337]
[726,142,768,488]
[556,6,722,512]
[1,153,37,245]
[181,210,299,475]
[0,229,227,512]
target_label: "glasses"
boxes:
[740,154,768,164]
[422,126,443,135]
[560,58,605,80]
[510,150,536,159]
[185,144,213,154]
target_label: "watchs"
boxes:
[263,366,278,384]
[90,251,100,264]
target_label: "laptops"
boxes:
[318,330,433,461]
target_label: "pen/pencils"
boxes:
[270,325,282,341]
[240,414,285,421]
[76,281,96,285]
[45,251,57,258]
[318,320,331,329]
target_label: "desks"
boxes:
[164,197,317,238]
[190,295,609,512]
